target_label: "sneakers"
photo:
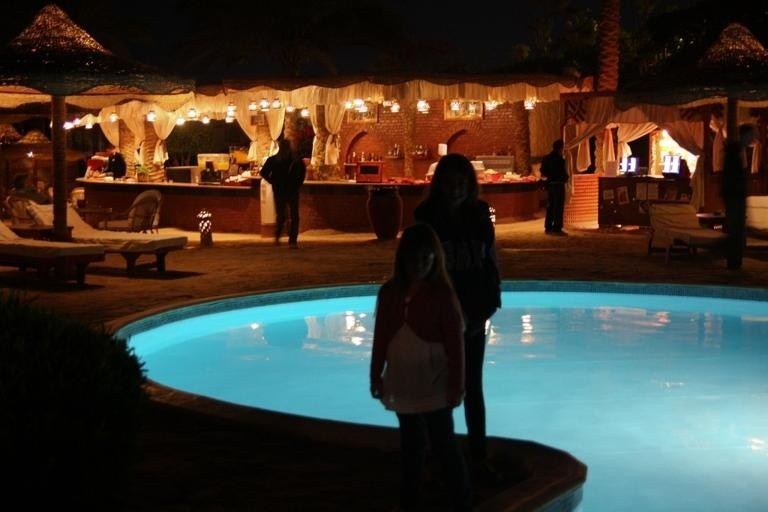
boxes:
[274,230,298,249]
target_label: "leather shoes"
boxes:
[544,229,568,237]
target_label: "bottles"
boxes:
[351,151,382,163]
[394,144,430,158]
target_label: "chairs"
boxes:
[644,200,727,268]
[0,189,189,287]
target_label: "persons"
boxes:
[719,125,754,274]
[371,225,472,512]
[11,174,50,204]
[260,140,306,249]
[71,160,87,187]
[413,155,500,478]
[103,145,126,177]
[540,140,569,236]
